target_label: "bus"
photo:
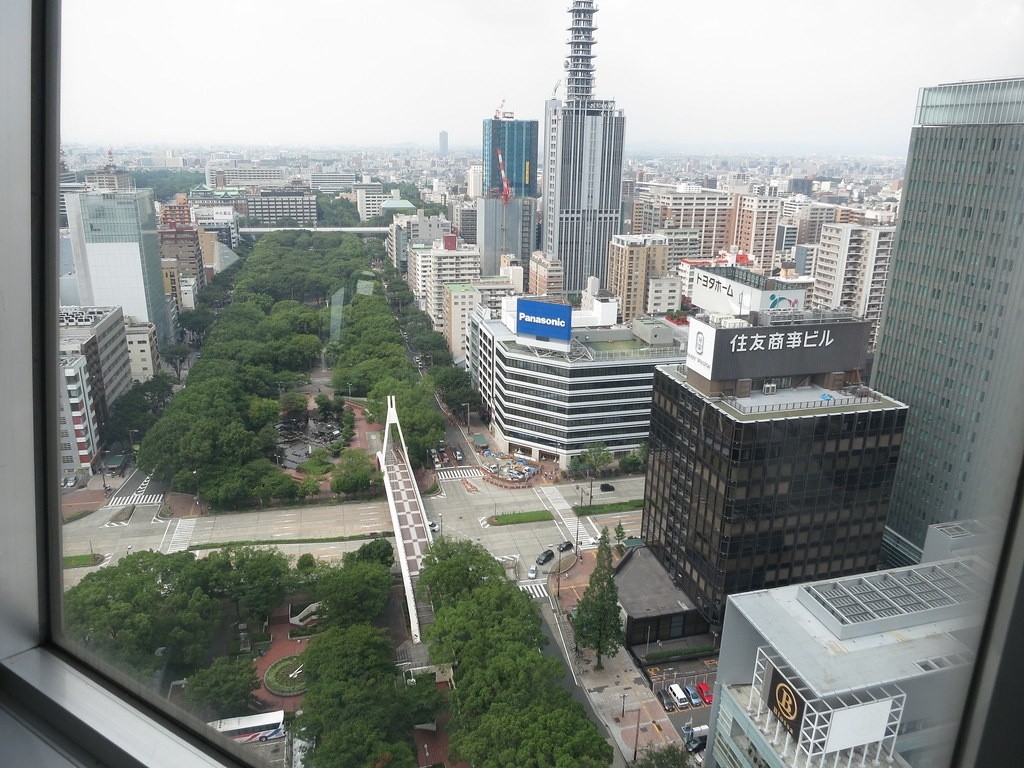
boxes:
[207,710,287,745]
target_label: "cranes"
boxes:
[491,148,511,205]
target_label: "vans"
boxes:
[669,683,689,709]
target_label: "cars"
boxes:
[528,565,538,579]
[403,332,423,369]
[274,417,341,444]
[428,521,439,532]
[476,433,537,482]
[430,438,462,468]
[686,735,707,753]
[60,477,78,487]
[535,549,554,565]
[557,540,573,552]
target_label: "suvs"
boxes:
[657,690,675,712]
[601,484,615,493]
[684,685,702,706]
[698,682,713,704]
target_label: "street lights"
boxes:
[438,513,443,537]
[619,694,633,720]
[461,403,471,435]
[347,383,352,397]
[130,429,139,451]
[276,381,282,394]
